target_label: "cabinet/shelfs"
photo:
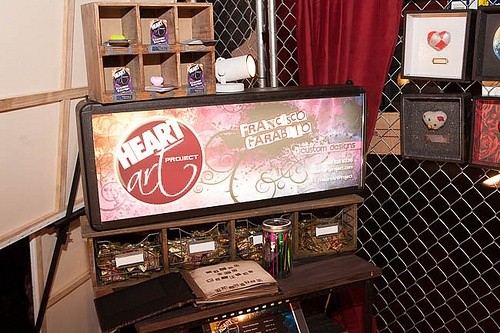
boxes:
[81,2,216,104]
[79,216,383,333]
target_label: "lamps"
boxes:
[215,54,256,92]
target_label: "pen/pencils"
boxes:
[264,232,290,279]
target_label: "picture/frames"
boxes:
[400,5,500,171]
